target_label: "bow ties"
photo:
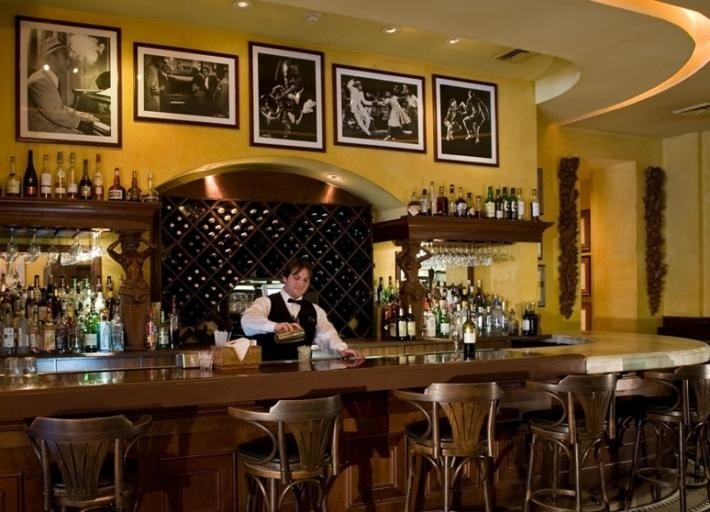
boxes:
[287,298,304,305]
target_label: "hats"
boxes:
[39,36,67,61]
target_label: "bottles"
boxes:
[466,192,474,217]
[522,304,530,336]
[158,310,169,350]
[143,173,159,202]
[108,168,126,200]
[510,188,517,219]
[0,273,124,356]
[463,308,476,360]
[436,186,448,217]
[398,307,407,340]
[127,170,143,201]
[5,156,21,196]
[530,189,540,221]
[502,187,510,219]
[388,305,398,341]
[53,151,66,198]
[78,159,93,199]
[419,189,431,216]
[406,194,421,216]
[145,303,158,351]
[168,297,179,350]
[407,304,416,340]
[495,189,503,219]
[530,302,538,336]
[455,187,467,217]
[377,277,384,304]
[39,154,52,197]
[67,152,78,199]
[23,149,38,197]
[517,188,525,221]
[474,196,484,218]
[484,186,496,218]
[429,181,437,216]
[425,279,518,339]
[92,153,104,200]
[382,308,389,340]
[386,276,394,301]
[448,184,456,216]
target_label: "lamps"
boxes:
[382,25,401,35]
[232,0,256,11]
[304,13,324,25]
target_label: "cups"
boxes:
[5,357,22,376]
[23,357,38,371]
[450,324,461,354]
[422,241,511,269]
[274,325,305,342]
[198,350,213,372]
[6,230,102,262]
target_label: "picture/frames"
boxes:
[133,41,240,130]
[247,40,327,154]
[13,13,124,148]
[431,73,500,168]
[331,62,428,154]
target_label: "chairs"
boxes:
[630,364,710,511]
[521,373,618,512]
[229,395,345,512]
[26,412,154,512]
[393,380,505,512]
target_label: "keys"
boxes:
[192,66,204,86]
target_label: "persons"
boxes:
[159,61,169,82]
[240,258,364,364]
[341,75,418,140]
[143,55,162,111]
[462,89,490,144]
[186,80,208,112]
[212,66,228,112]
[445,97,462,141]
[27,36,99,134]
[267,60,316,137]
[201,67,217,91]
